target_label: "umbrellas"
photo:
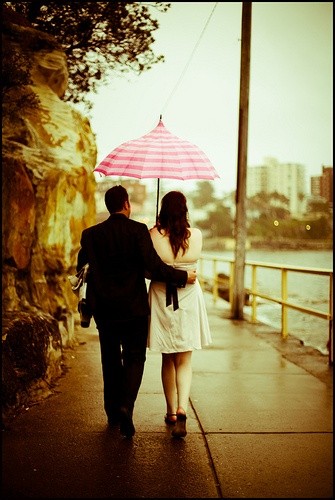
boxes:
[91,114,221,226]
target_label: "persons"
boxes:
[148,191,212,436]
[76,185,197,436]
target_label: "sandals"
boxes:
[165,412,177,426]
[171,408,187,439]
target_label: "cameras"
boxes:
[78,298,92,328]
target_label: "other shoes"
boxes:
[118,407,136,438]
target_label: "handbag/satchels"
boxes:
[67,263,92,328]
